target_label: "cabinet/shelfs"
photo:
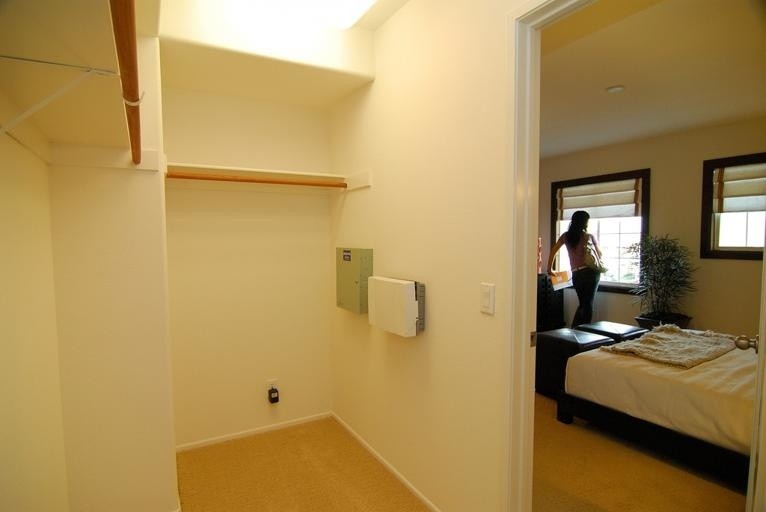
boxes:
[537,272,564,332]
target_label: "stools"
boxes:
[575,320,650,344]
[535,327,615,402]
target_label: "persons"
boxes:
[546,211,608,328]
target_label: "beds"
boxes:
[557,329,759,497]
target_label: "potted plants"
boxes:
[627,233,703,331]
